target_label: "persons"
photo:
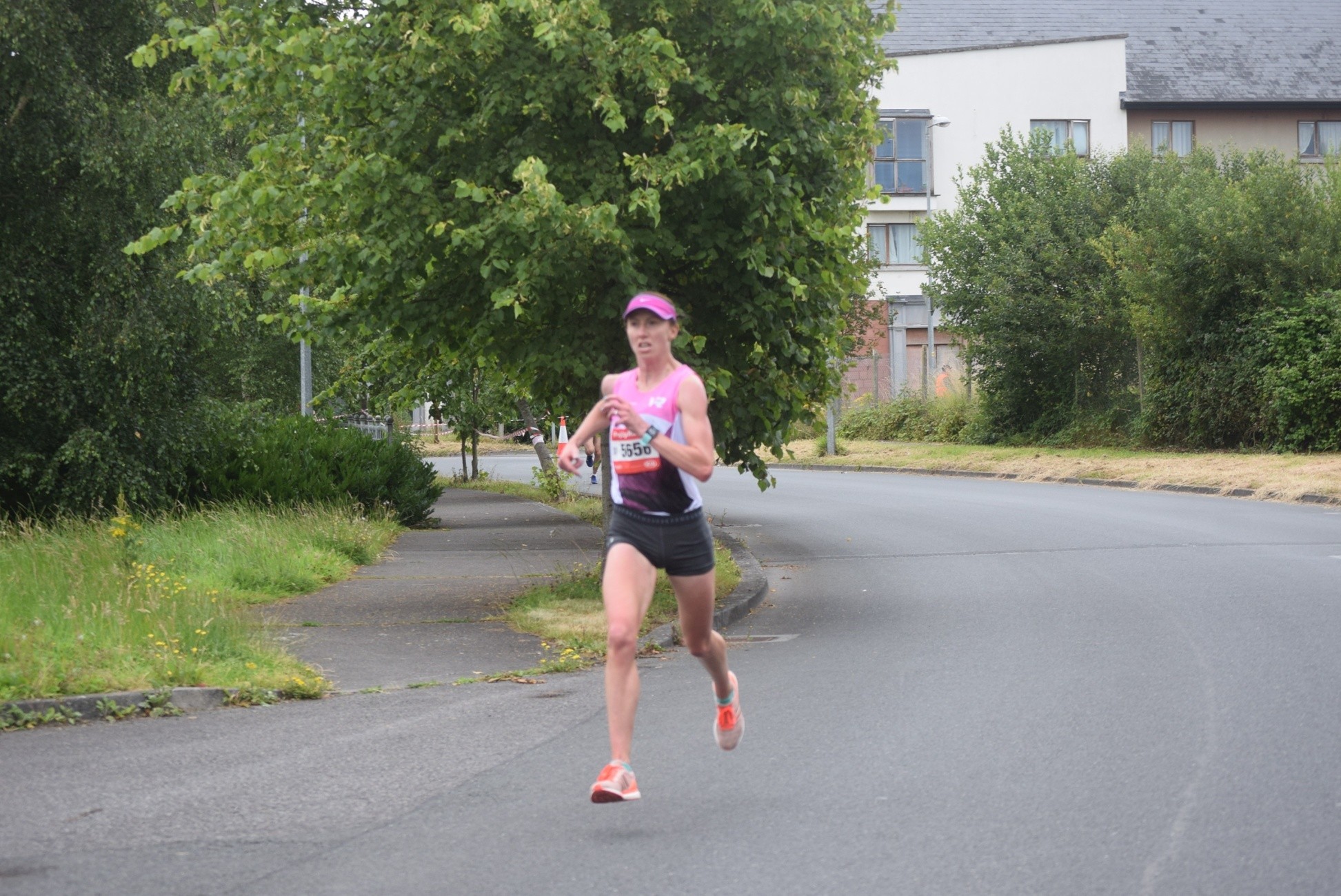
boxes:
[558,290,742,803]
[583,433,601,483]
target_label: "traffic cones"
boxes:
[552,417,569,458]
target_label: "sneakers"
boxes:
[586,455,593,467]
[590,764,641,803]
[711,670,744,751]
[590,474,596,484]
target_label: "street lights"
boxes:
[923,119,950,401]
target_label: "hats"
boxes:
[623,295,677,324]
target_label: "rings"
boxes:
[615,409,618,415]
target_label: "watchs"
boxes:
[641,425,661,445]
